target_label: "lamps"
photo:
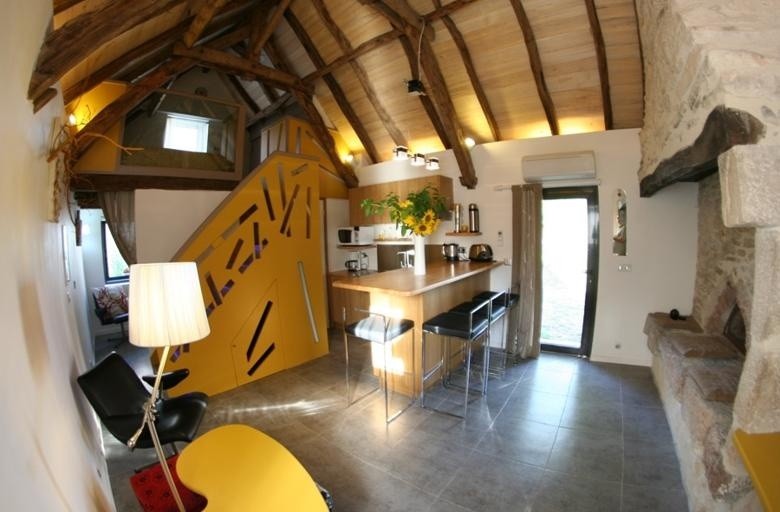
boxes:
[394,18,440,171]
[126,261,211,512]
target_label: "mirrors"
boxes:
[611,188,627,256]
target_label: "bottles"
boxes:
[468,203,479,232]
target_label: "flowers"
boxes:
[360,184,448,238]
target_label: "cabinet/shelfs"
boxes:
[327,269,381,330]
[348,175,453,226]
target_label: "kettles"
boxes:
[442,243,458,261]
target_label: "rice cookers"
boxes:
[469,243,493,261]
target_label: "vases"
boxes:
[413,233,426,276]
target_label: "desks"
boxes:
[175,423,329,512]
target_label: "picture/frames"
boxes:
[62,224,71,282]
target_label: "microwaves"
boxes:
[336,226,374,243]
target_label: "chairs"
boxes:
[77,351,210,472]
[341,284,521,423]
[91,287,129,350]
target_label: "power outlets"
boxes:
[457,247,466,254]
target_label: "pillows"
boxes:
[92,284,129,316]
